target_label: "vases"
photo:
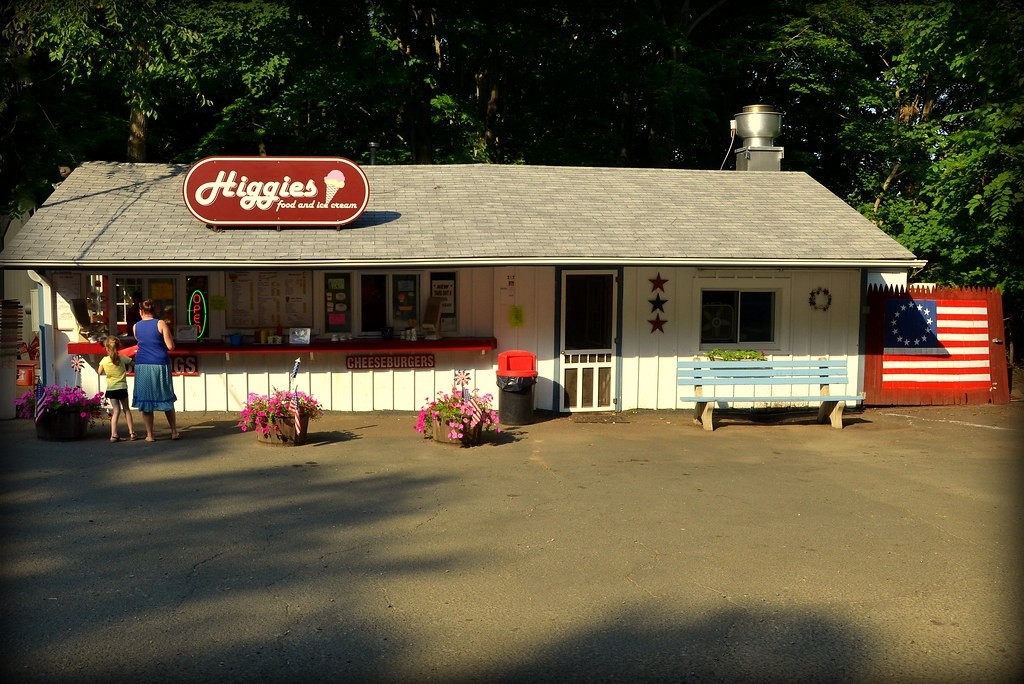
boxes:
[34,405,86,440]
[257,412,312,445]
[430,411,483,444]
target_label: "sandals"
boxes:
[110,436,120,442]
[129,433,137,441]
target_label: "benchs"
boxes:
[676,357,863,432]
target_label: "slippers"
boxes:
[172,433,179,439]
[146,436,155,442]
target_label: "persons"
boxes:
[133,299,180,442]
[98,334,139,442]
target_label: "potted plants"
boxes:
[704,349,768,375]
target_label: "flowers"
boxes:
[13,384,114,432]
[413,386,501,440]
[238,386,324,436]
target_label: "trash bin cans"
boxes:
[496,350,538,426]
